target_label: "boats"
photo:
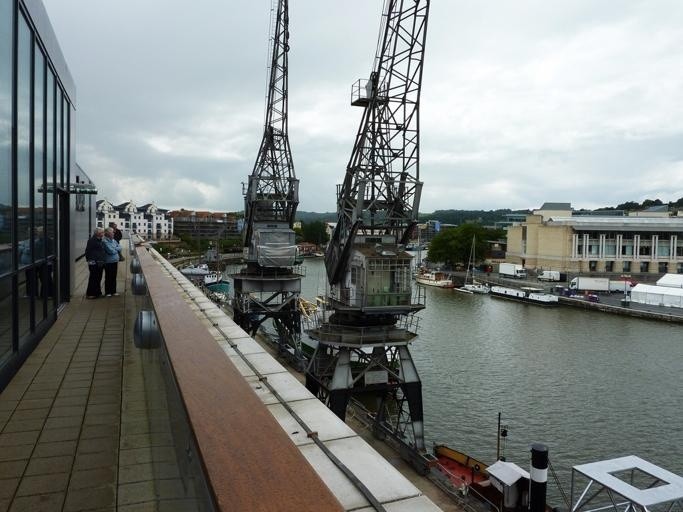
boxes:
[414,265,454,288]
[203,270,222,285]
[489,285,561,308]
[411,226,422,280]
[179,263,209,275]
[452,231,490,294]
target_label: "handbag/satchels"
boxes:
[118,251,125,261]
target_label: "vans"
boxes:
[537,270,561,282]
[479,264,492,272]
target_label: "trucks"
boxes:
[497,262,527,279]
[609,280,634,292]
[568,276,611,294]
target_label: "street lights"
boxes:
[620,273,631,306]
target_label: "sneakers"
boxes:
[106,294,111,297]
[113,293,120,296]
[85,295,94,299]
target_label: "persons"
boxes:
[81,228,103,302]
[102,229,125,300]
[102,221,126,265]
